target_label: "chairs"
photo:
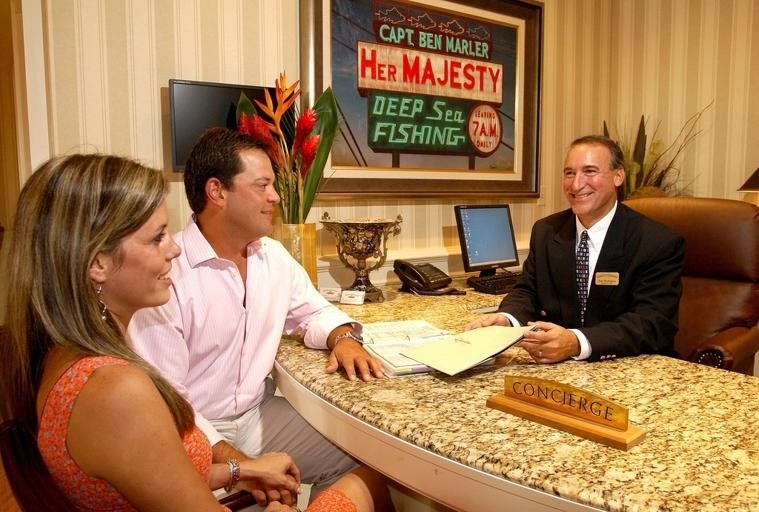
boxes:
[621,196,759,375]
[1,420,257,512]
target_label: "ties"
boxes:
[575,230,589,324]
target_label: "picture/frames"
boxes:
[297,0,544,206]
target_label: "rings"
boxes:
[538,352,543,358]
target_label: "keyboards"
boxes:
[466,272,522,295]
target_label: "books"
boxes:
[356,320,536,378]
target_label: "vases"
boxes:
[269,224,319,293]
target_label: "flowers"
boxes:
[235,69,344,224]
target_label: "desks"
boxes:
[273,271,759,512]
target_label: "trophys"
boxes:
[319,211,404,302]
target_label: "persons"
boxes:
[0,127,396,512]
[464,134,686,365]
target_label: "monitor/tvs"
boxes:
[168,79,295,173]
[454,204,520,276]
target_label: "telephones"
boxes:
[393,260,452,291]
